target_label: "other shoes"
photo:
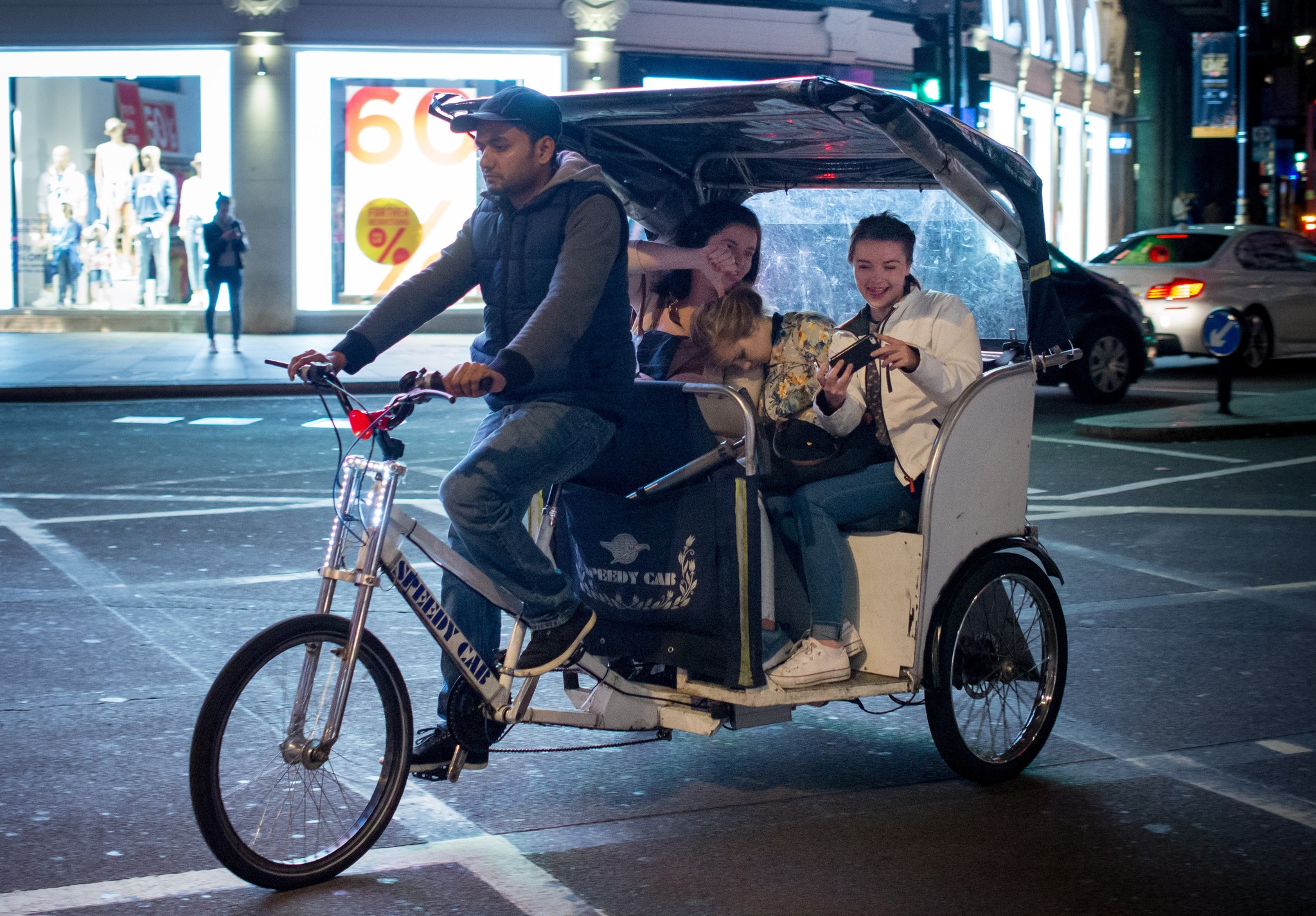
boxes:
[230,340,242,354]
[207,340,218,353]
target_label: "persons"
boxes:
[287,87,637,771]
[35,117,203,305]
[764,210,983,690]
[689,281,841,672]
[629,201,764,413]
[203,192,248,354]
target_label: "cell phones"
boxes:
[828,334,882,380]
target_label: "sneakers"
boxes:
[378,724,490,772]
[513,599,596,677]
[761,619,865,688]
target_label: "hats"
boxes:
[450,84,564,143]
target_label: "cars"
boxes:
[1087,227,1152,265]
[1079,225,1315,372]
[1017,230,1155,402]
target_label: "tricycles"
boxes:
[185,73,1075,888]
